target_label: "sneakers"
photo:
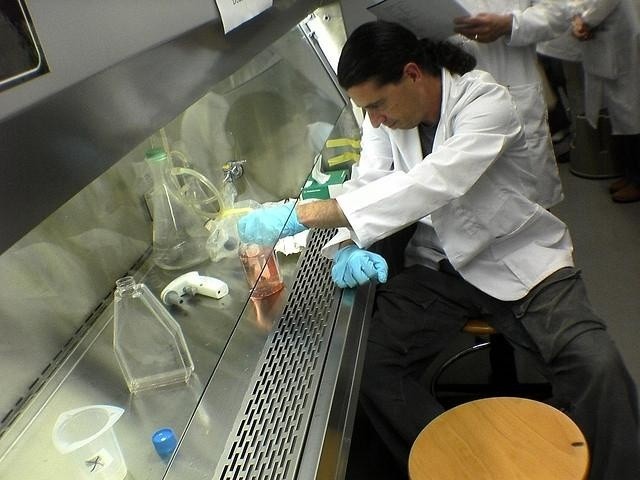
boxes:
[612,178,640,203]
[552,127,570,142]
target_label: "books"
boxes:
[364,0,473,42]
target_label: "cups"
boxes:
[51,406,127,479]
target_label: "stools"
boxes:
[407,320,592,480]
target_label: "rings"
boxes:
[475,34,479,41]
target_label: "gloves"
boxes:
[237,203,309,245]
[331,245,389,288]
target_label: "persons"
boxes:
[440,0,572,214]
[565,0,640,204]
[532,0,588,160]
[233,19,640,479]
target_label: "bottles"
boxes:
[145,149,225,270]
[113,276,194,393]
[240,241,283,299]
